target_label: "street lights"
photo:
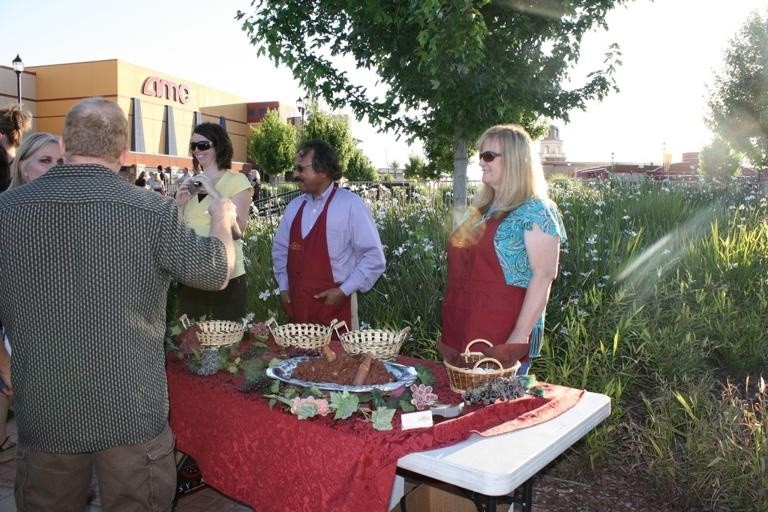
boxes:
[7,52,24,108]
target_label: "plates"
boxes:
[266,355,410,393]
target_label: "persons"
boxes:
[0,105,33,193]
[175,122,256,325]
[438,124,568,373]
[240,162,254,185]
[269,139,387,338]
[0,130,64,463]
[0,97,238,512]
[136,163,193,198]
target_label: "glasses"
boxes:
[191,141,216,150]
[480,152,501,161]
[294,164,313,172]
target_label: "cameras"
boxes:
[185,180,202,195]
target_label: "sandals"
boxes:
[0,437,16,462]
